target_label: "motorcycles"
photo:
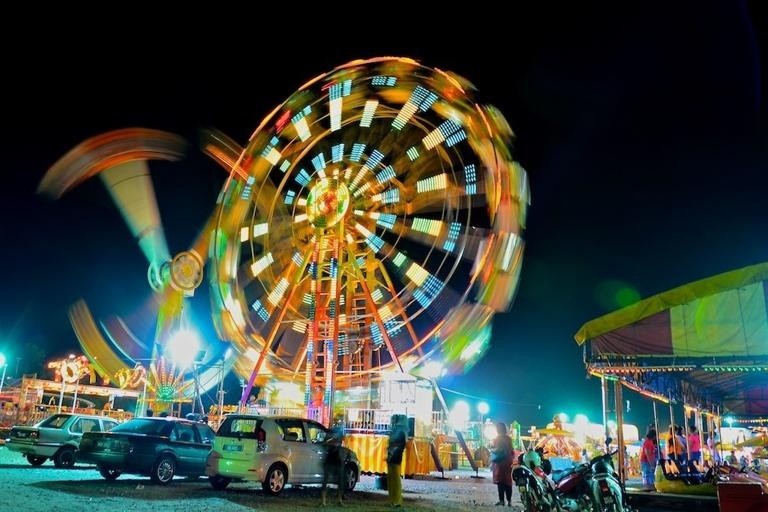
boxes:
[511,437,638,512]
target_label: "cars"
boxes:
[5,413,215,486]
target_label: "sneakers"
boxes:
[508,503,512,506]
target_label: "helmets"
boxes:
[591,460,613,476]
[523,450,541,468]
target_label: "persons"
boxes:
[580,448,590,463]
[625,420,762,493]
[317,413,349,510]
[381,414,408,508]
[488,422,514,506]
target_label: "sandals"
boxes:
[495,501,505,506]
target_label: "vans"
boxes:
[205,413,362,496]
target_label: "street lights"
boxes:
[727,417,733,428]
[455,401,467,466]
[171,332,196,417]
[478,402,487,468]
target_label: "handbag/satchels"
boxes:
[491,450,505,462]
